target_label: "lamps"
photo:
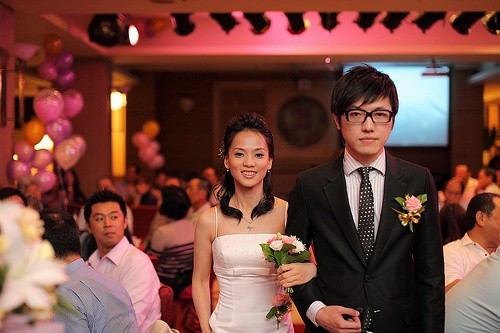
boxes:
[89,14,139,48]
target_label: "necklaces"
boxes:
[232,196,265,230]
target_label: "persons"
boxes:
[145,187,196,299]
[431,163,500,241]
[286,62,446,333]
[84,191,162,333]
[33,210,140,333]
[0,164,229,262]
[443,192,500,294]
[444,253,500,333]
[192,112,317,333]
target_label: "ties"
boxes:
[356,167,377,333]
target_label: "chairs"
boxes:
[159,281,202,333]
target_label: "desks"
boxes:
[131,205,156,239]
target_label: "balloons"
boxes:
[10,34,88,192]
[130,121,166,170]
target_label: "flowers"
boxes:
[258,232,311,330]
[392,194,427,233]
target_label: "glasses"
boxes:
[340,108,394,124]
